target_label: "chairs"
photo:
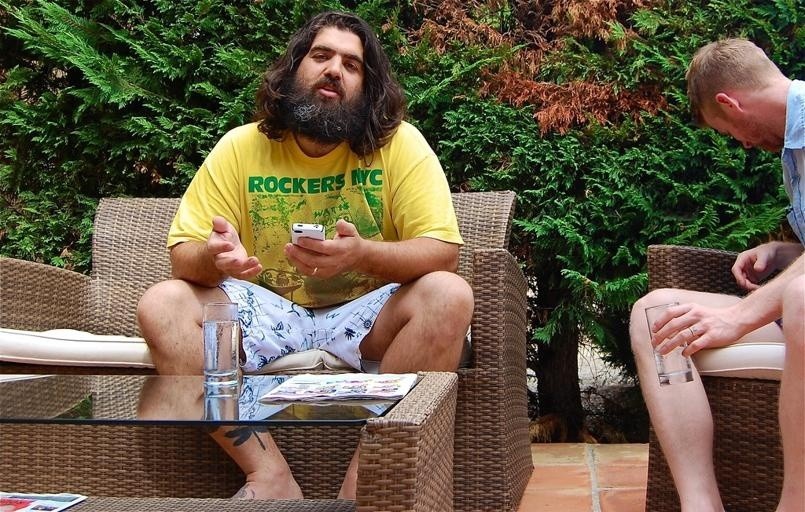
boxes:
[0,189,536,510]
[640,243,782,512]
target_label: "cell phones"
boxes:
[292,222,325,245]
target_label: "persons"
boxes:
[627,37,805,511]
[136,11,475,501]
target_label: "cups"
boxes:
[644,302,699,387]
[201,301,239,388]
[203,387,240,423]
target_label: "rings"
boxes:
[311,267,318,276]
[688,325,699,338]
